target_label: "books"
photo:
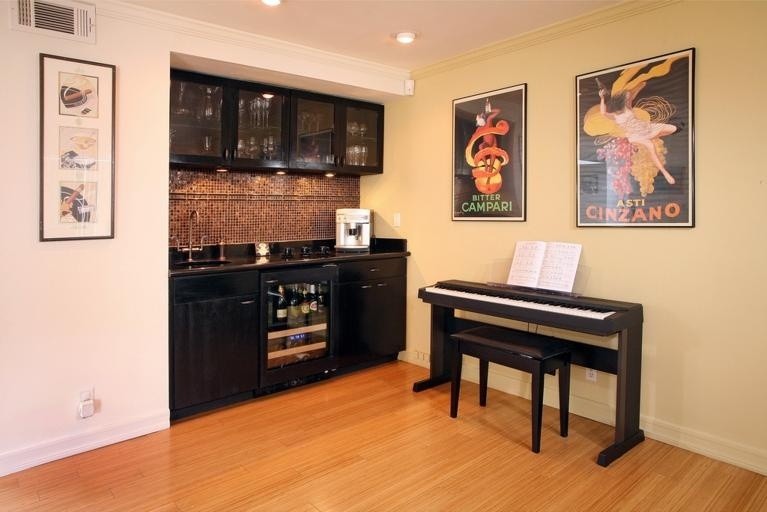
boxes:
[507,239,582,294]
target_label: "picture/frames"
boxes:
[38,53,116,243]
[450,46,696,228]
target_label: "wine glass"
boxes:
[238,95,369,167]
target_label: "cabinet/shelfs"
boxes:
[170,267,258,424]
[169,67,384,178]
[257,264,337,395]
[337,256,407,376]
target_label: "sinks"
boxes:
[172,261,232,268]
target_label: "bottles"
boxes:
[198,135,218,155]
[173,79,215,124]
[272,282,326,327]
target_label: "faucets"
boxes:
[170,209,209,261]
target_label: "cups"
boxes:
[301,246,312,254]
[319,246,329,253]
[285,247,294,256]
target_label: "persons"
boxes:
[595,90,678,187]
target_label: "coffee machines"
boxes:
[334,207,373,252]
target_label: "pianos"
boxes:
[413,280,645,467]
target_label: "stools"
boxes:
[448,325,570,453]
